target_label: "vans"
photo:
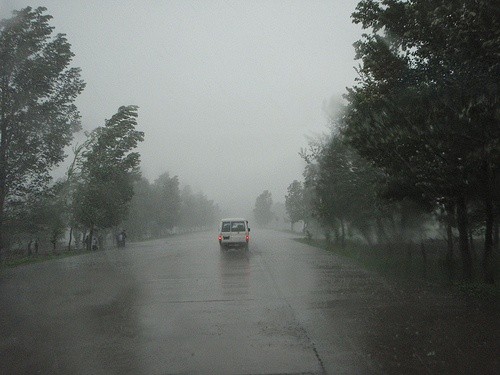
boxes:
[218,218,250,251]
